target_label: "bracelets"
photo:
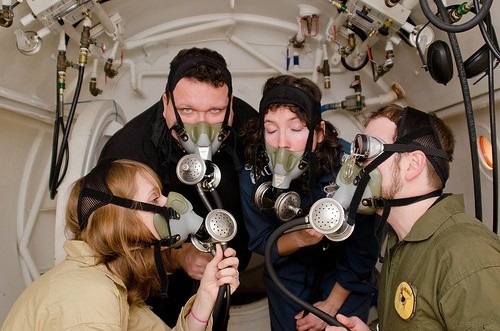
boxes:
[190,309,210,323]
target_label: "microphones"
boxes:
[473,61,500,85]
[416,4,500,86]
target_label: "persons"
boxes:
[240,76,392,331]
[0,159,240,331]
[324,104,500,331]
[97,47,270,331]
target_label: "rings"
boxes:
[235,269,239,277]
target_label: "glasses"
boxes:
[349,133,450,162]
